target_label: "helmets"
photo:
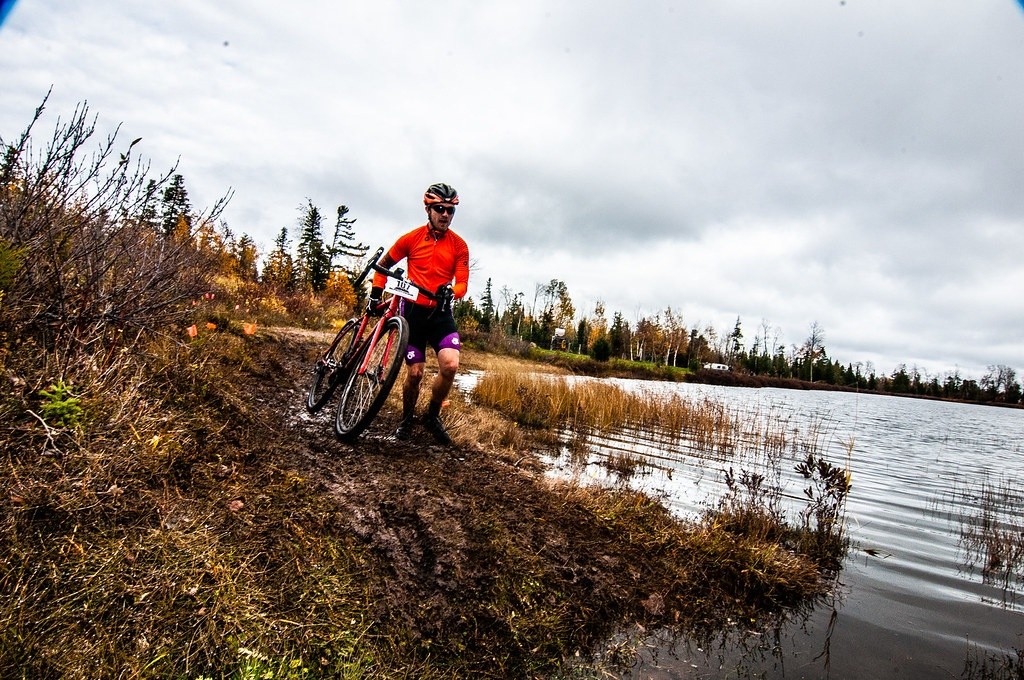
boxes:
[424,183,459,206]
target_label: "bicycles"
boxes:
[305,247,445,440]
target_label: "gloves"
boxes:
[435,285,456,304]
[365,287,383,317]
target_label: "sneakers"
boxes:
[395,417,413,439]
[423,413,450,443]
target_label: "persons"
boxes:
[365,183,470,448]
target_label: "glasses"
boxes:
[431,205,455,215]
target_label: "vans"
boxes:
[704,362,729,371]
